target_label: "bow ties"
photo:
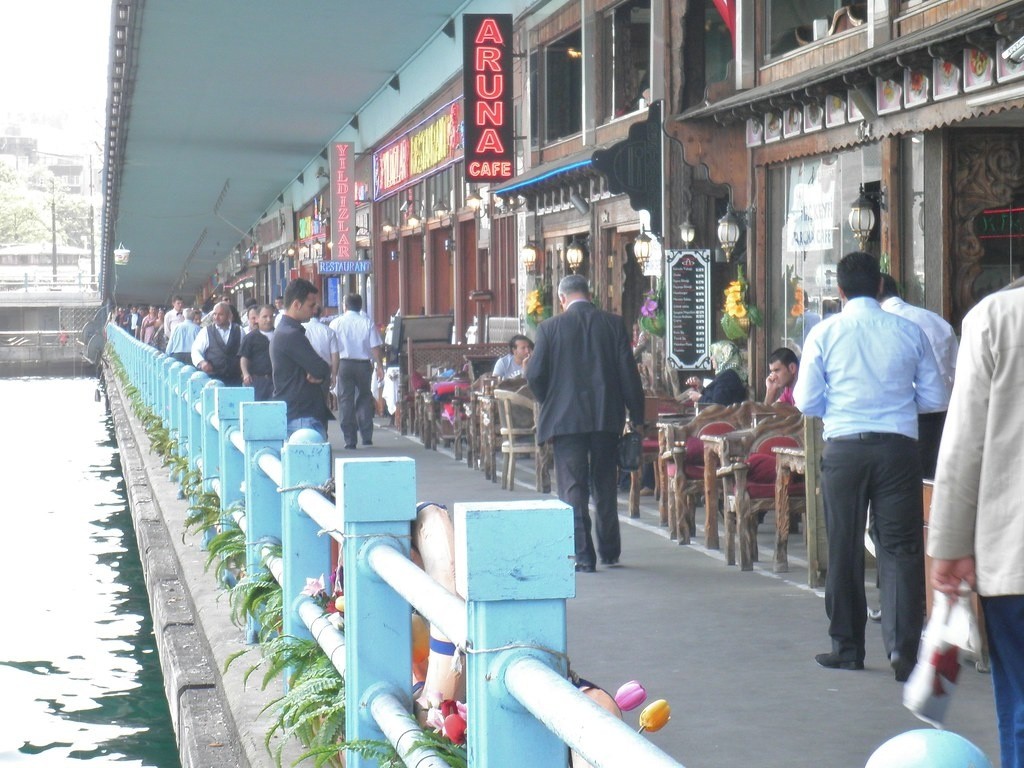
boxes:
[177,312,182,316]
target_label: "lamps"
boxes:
[718,201,757,261]
[352,186,525,242]
[521,236,539,276]
[848,182,888,251]
[679,212,695,250]
[567,235,592,275]
[634,224,664,269]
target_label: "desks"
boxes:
[477,394,500,478]
[772,448,803,569]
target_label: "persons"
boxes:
[794,248,958,681]
[760,347,805,417]
[491,334,534,382]
[529,273,647,575]
[927,251,1024,766]
[108,276,385,450]
[685,336,748,408]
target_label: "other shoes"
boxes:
[815,654,865,670]
[601,556,619,564]
[343,444,357,449]
[362,440,373,445]
[890,650,916,682]
[575,562,597,573]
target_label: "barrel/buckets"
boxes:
[114,242,130,266]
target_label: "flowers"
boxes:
[724,281,749,317]
[642,291,659,317]
[526,290,546,317]
[428,681,672,752]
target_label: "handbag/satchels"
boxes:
[617,433,642,473]
[901,578,974,731]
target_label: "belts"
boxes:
[828,432,918,442]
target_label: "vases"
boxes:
[530,313,544,324]
[730,316,750,346]
[645,316,665,337]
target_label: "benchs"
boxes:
[396,343,827,587]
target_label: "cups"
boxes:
[902,634,967,720]
